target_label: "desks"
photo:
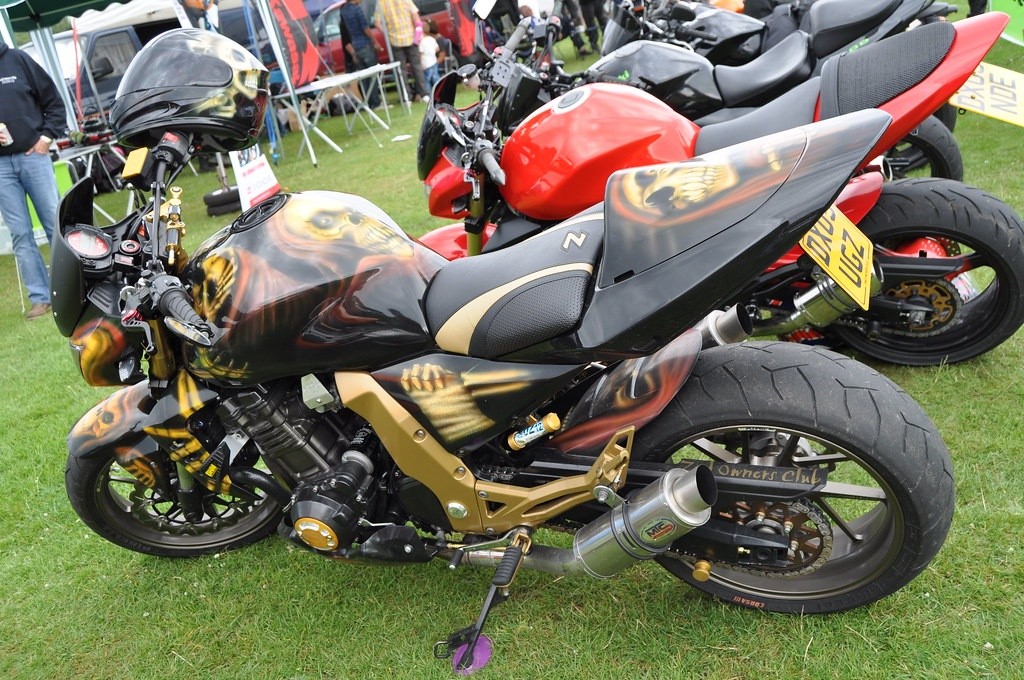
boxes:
[271,61,412,155]
[45,139,126,205]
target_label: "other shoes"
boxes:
[27,303,53,320]
[578,48,594,55]
[372,102,395,112]
[421,95,430,103]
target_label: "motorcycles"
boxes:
[397,16,1024,368]
[45,26,953,677]
[518,3,977,181]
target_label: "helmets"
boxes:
[109,28,270,155]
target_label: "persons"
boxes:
[1,33,67,319]
[965,0,989,18]
[341,0,393,111]
[429,20,450,71]
[510,0,610,57]
[338,3,366,109]
[375,0,432,107]
[418,22,442,98]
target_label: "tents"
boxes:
[1,0,318,186]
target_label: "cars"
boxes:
[300,2,496,87]
